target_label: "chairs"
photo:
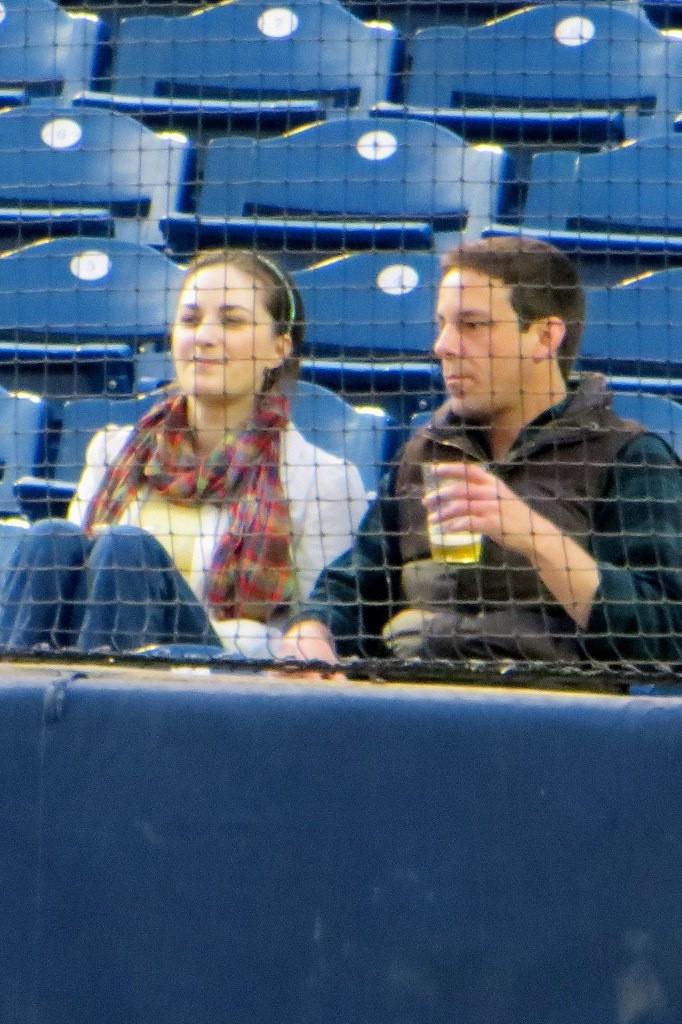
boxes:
[0,0,682,519]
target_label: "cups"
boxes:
[419,460,488,565]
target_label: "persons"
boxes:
[0,247,371,679]
[268,234,682,695]
